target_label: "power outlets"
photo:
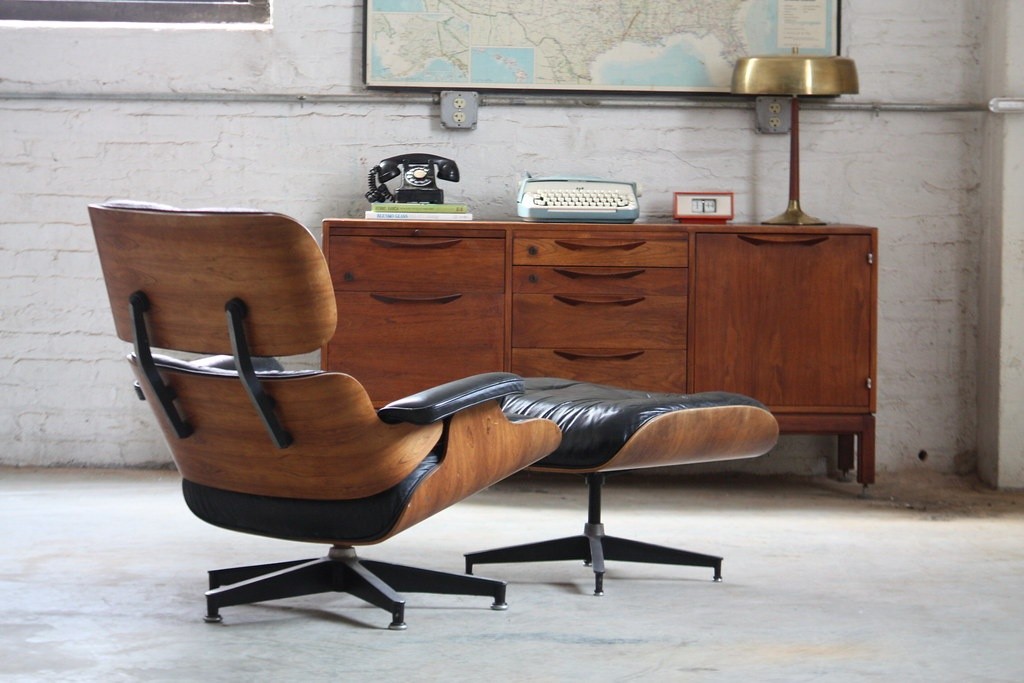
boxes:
[755,97,792,133]
[440,91,478,130]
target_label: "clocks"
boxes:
[673,191,734,224]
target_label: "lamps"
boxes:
[730,55,860,225]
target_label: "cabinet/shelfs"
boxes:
[508,222,691,393]
[690,223,880,500]
[323,218,506,411]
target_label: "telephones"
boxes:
[379,154,460,206]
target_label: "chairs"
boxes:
[86,197,563,630]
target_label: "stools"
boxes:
[462,376,780,597]
